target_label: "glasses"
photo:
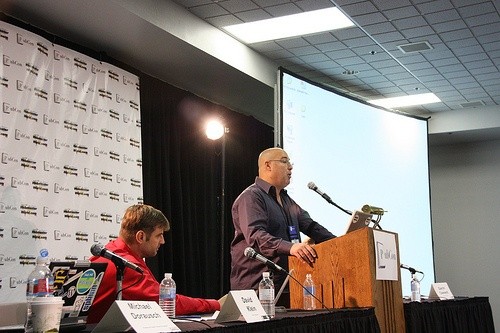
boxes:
[269,159,294,165]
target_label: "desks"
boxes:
[404,295,496,332]
[0,305,381,333]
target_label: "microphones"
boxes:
[400,265,417,272]
[244,247,286,273]
[91,242,143,273]
[308,182,331,202]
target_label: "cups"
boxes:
[29,297,65,333]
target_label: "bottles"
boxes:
[411,277,420,302]
[259,273,275,318]
[303,274,315,310]
[26,257,56,333]
[159,273,176,319]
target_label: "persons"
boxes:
[86,204,229,326]
[229,148,340,308]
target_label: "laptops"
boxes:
[274,269,294,305]
[0,262,107,333]
[346,211,373,234]
